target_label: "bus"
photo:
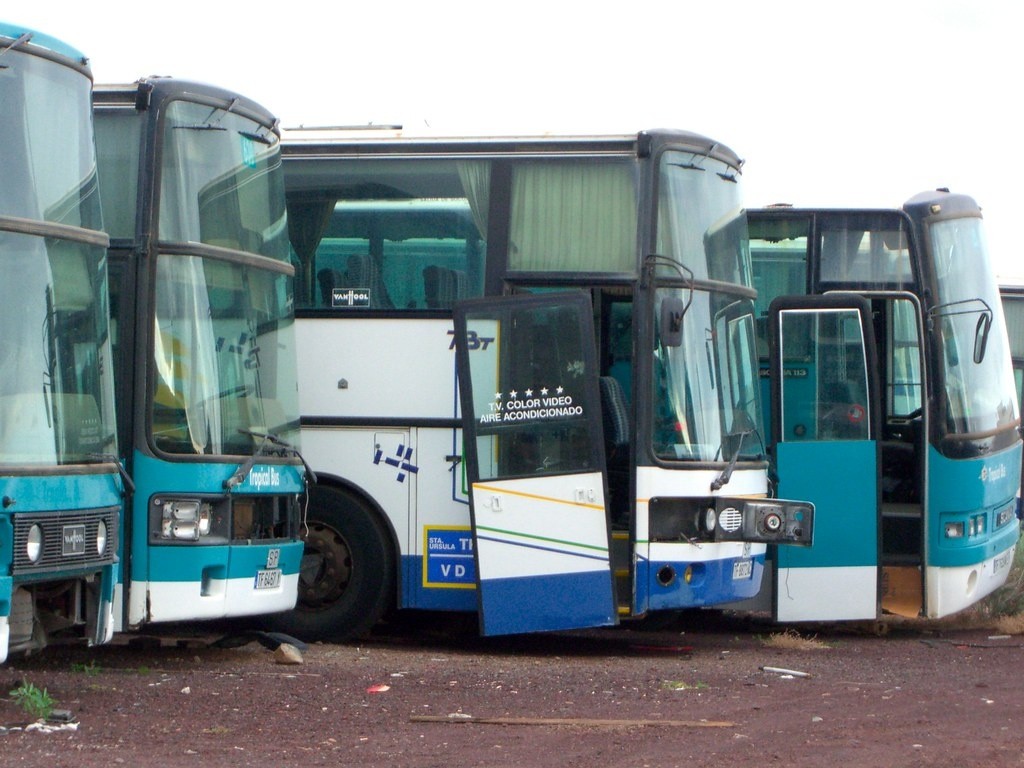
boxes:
[317,182,1023,632]
[90,77,318,654]
[1,22,138,672]
[261,126,817,645]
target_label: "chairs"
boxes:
[317,268,349,310]
[422,265,455,311]
[348,253,383,311]
[451,268,472,311]
